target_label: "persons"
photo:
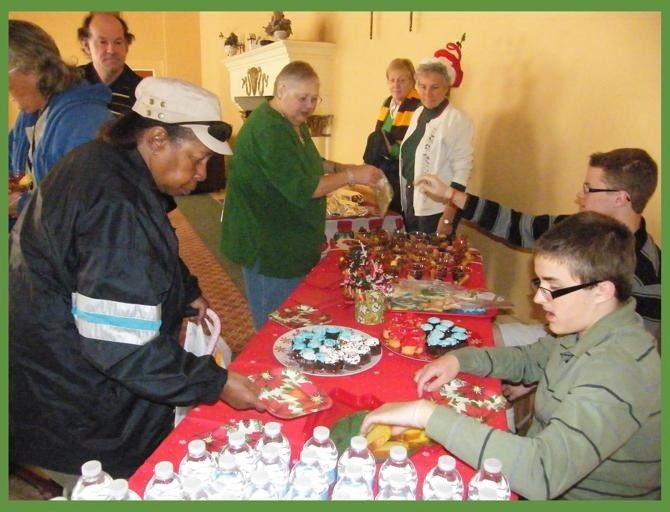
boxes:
[358,210,661,500]
[396,64,474,238]
[8,19,124,217]
[68,12,146,135]
[364,59,427,220]
[10,75,265,494]
[221,60,387,335]
[412,148,661,401]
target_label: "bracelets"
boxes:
[333,161,339,173]
[345,166,356,187]
[442,185,455,205]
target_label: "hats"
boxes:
[132,76,233,156]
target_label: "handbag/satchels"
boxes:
[362,131,400,178]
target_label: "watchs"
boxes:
[438,216,454,228]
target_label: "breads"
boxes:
[291,327,380,370]
[383,317,468,356]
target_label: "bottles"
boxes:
[248,34,256,50]
[65,421,512,501]
[240,42,245,54]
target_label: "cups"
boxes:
[356,230,473,289]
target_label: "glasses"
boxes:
[169,120,232,143]
[530,278,605,301]
[582,183,632,202]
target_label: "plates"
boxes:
[246,369,332,419]
[272,323,383,376]
[420,377,508,424]
[331,409,430,463]
[380,317,487,362]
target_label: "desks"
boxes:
[320,197,406,261]
[123,244,523,502]
